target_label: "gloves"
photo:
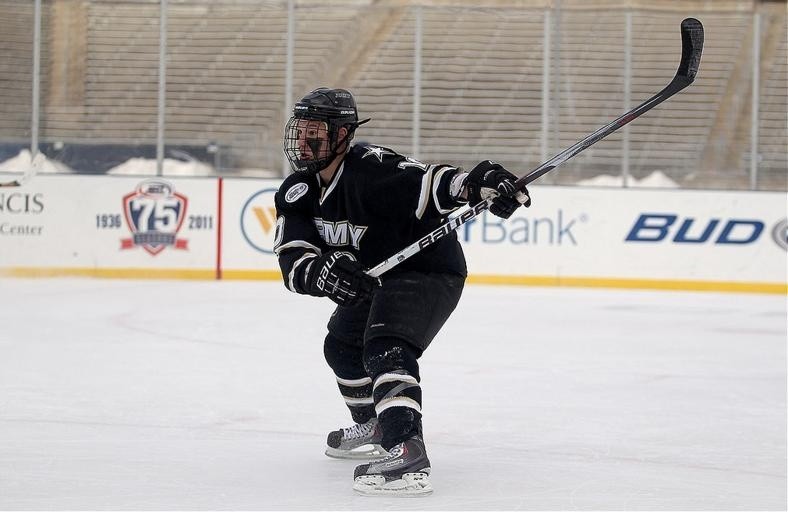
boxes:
[306,250,381,309]
[466,159,531,218]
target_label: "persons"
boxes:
[269,86,531,484]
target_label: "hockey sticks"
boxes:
[365,17,704,277]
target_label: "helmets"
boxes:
[284,88,358,175]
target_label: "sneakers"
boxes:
[355,433,431,482]
[327,417,385,450]
